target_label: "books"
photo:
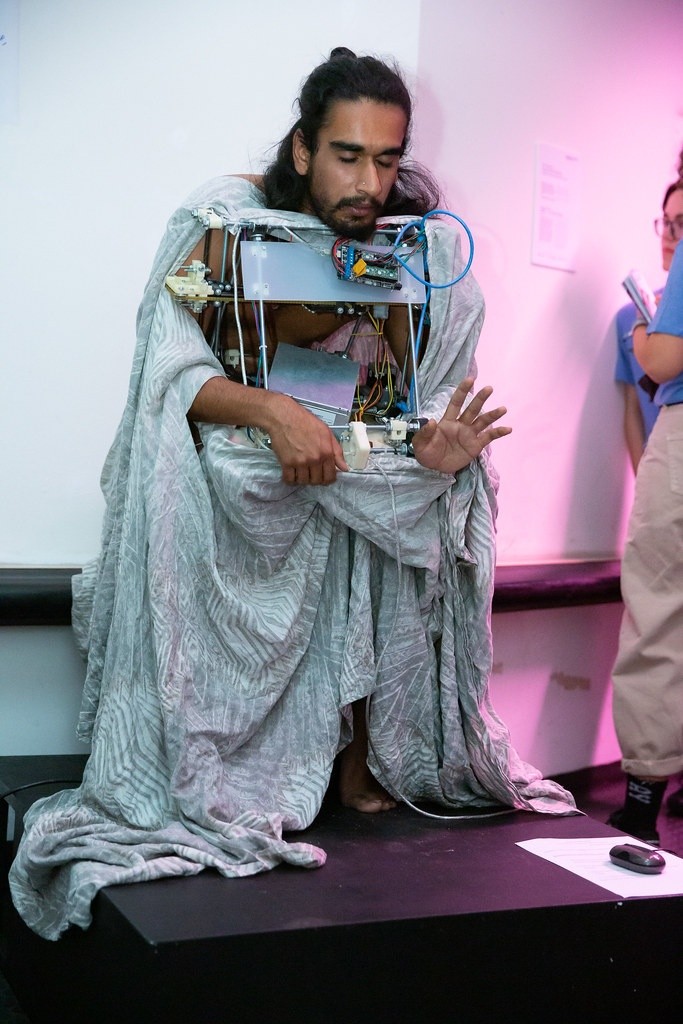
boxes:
[622,269,658,326]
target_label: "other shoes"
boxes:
[605,810,660,845]
[667,789,683,814]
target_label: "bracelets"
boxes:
[632,319,647,333]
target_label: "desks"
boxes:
[0,753,683,1024]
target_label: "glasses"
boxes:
[654,217,683,240]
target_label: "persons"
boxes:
[608,149,683,848]
[8,46,584,941]
